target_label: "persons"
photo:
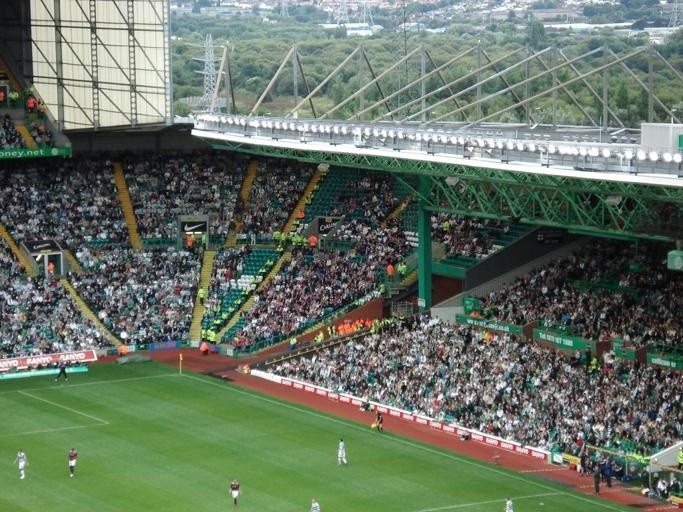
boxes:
[1,65,57,148]
[12,446,30,480]
[67,445,78,479]
[229,478,241,506]
[1,149,240,381]
[242,152,683,512]
